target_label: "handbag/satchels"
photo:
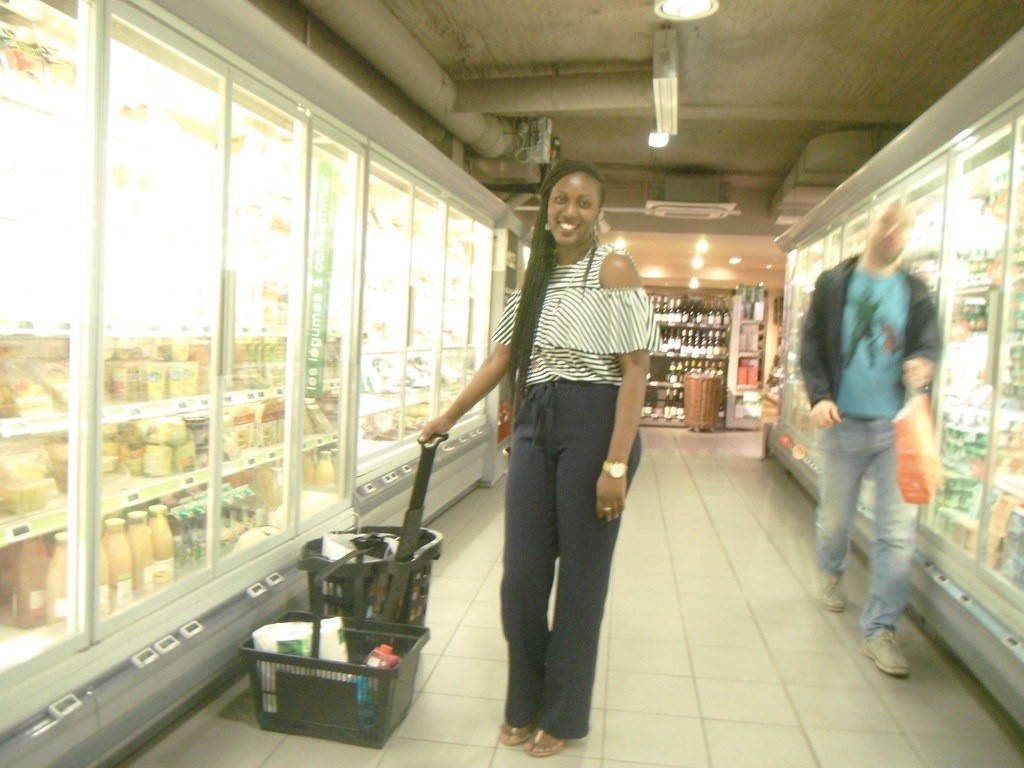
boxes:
[893,393,941,503]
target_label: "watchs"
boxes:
[600,462,629,478]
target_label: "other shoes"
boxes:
[500,714,542,745]
[524,728,566,757]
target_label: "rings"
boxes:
[603,507,612,511]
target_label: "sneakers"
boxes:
[820,565,847,611]
[861,626,911,674]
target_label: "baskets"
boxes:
[238,563,430,748]
[683,373,720,432]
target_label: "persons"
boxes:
[801,204,941,677]
[421,158,662,757]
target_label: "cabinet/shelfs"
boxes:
[638,321,730,430]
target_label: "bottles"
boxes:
[645,389,654,413]
[126,510,156,598]
[664,389,685,418]
[302,447,338,492]
[0,530,67,630]
[660,328,727,355]
[651,295,730,325]
[668,360,725,384]
[99,517,134,620]
[148,504,175,588]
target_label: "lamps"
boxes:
[654,0,720,21]
